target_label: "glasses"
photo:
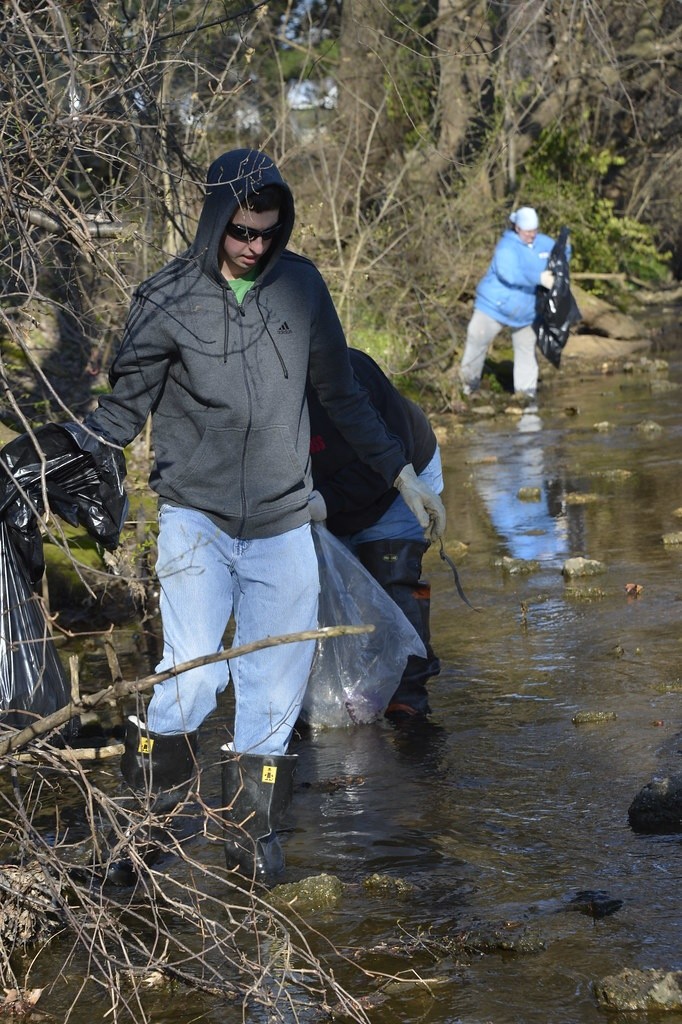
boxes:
[226,221,281,243]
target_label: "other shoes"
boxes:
[463,384,481,399]
[524,400,538,414]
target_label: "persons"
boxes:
[456,208,572,398]
[67,145,446,888]
[309,346,445,729]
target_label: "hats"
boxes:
[509,207,539,229]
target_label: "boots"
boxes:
[362,540,442,721]
[219,744,302,888]
[73,714,200,886]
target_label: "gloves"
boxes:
[540,270,555,290]
[393,460,446,543]
